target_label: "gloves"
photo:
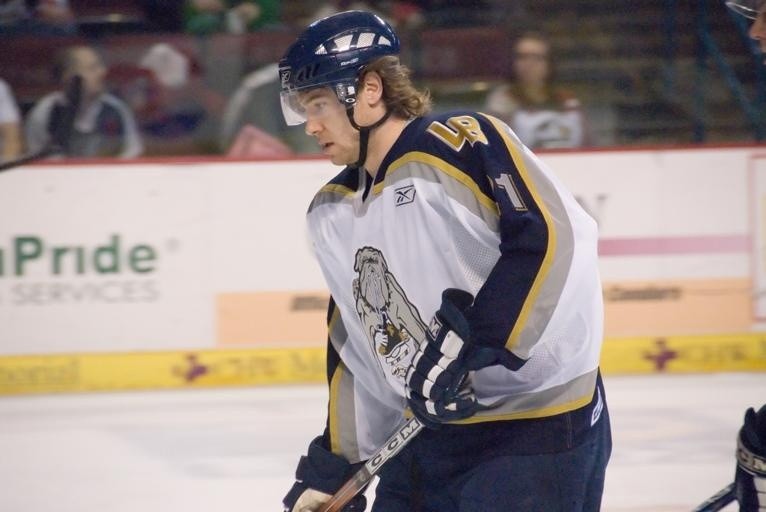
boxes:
[404,286,480,431]
[281,436,368,512]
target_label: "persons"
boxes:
[0,80,23,164]
[728,1,766,511]
[485,32,587,156]
[3,0,75,27]
[25,44,146,161]
[215,65,321,157]
[274,9,613,509]
[179,0,282,35]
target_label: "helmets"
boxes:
[278,10,402,126]
